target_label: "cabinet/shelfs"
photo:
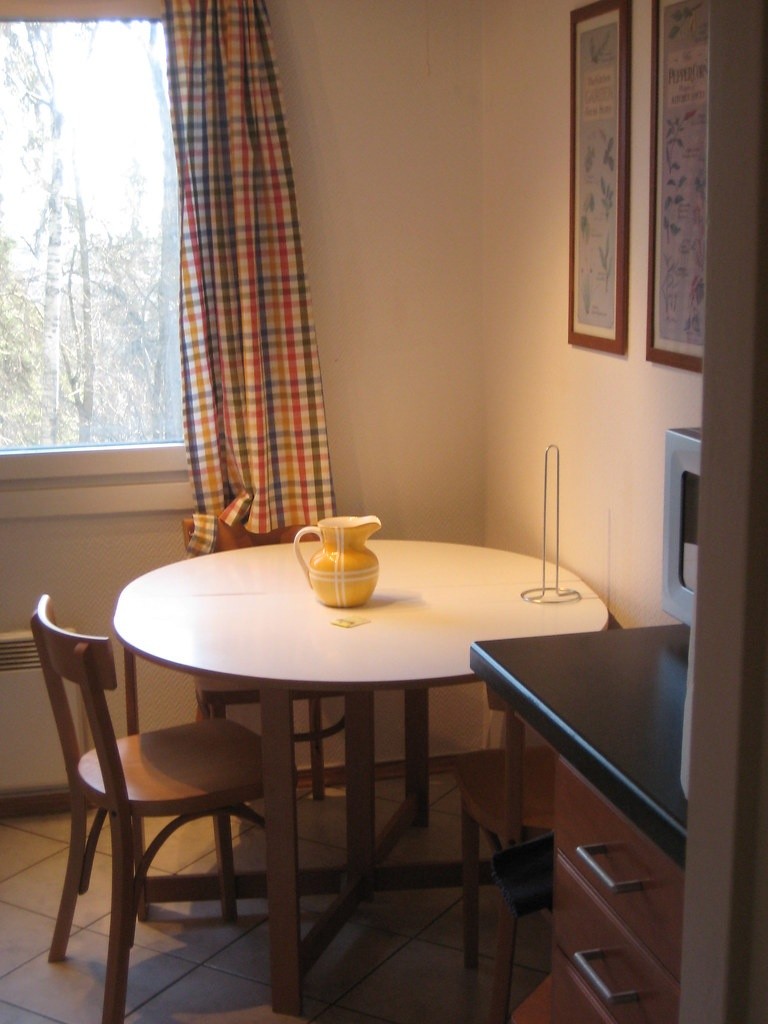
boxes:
[470,624,691,1024]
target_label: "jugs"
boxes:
[293,515,382,607]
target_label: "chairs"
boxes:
[182,515,351,799]
[462,684,623,1024]
[32,592,266,1024]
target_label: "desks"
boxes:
[113,541,610,1013]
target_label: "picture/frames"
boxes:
[644,1,716,373]
[565,0,634,356]
